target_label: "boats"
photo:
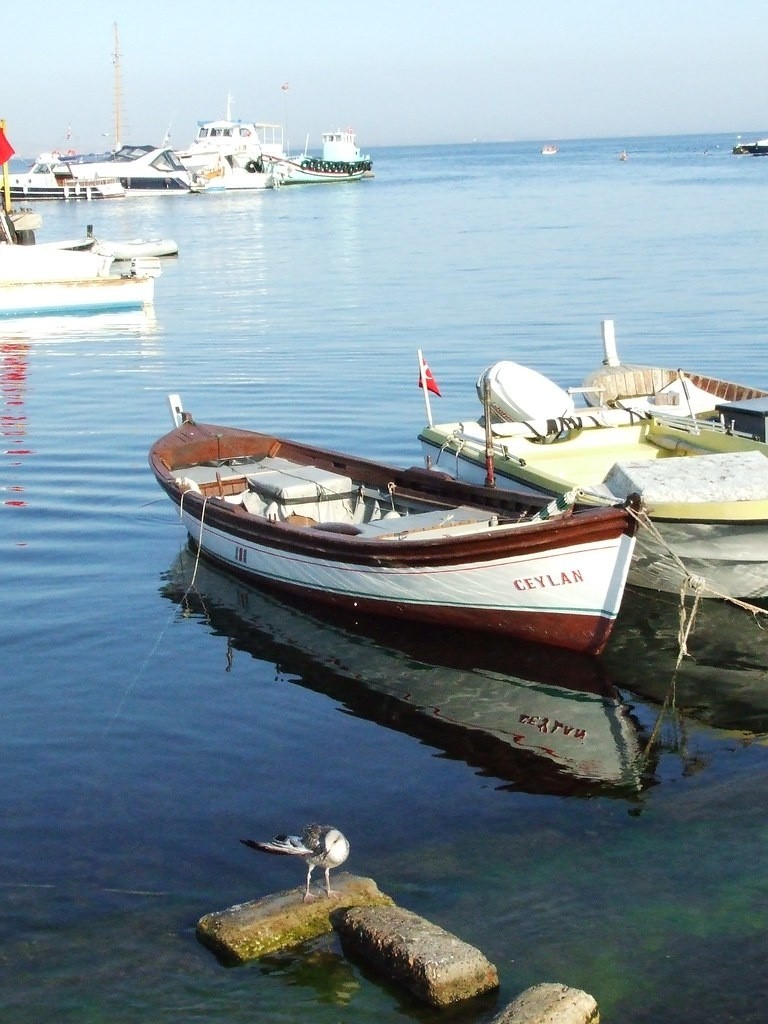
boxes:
[146,393,655,655]
[0,87,376,316]
[739,138,768,154]
[541,145,559,155]
[414,317,768,608]
[159,533,655,799]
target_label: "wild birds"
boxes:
[239,822,350,904]
